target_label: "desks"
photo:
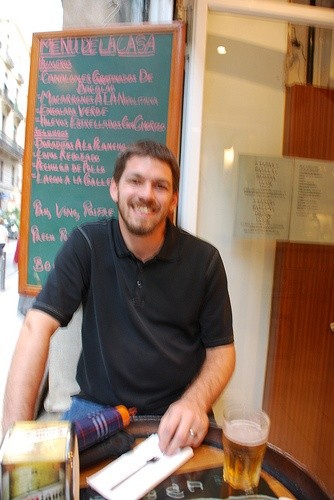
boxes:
[80,416,329,500]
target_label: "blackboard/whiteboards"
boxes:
[17,22,185,297]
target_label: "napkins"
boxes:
[86,432,193,500]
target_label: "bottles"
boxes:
[73,405,137,452]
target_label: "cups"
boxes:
[222,405,271,491]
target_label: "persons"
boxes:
[3,141,236,456]
[0,219,19,252]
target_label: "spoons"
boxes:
[110,457,159,491]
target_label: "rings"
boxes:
[189,429,197,437]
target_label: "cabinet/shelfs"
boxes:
[263,241,334,499]
